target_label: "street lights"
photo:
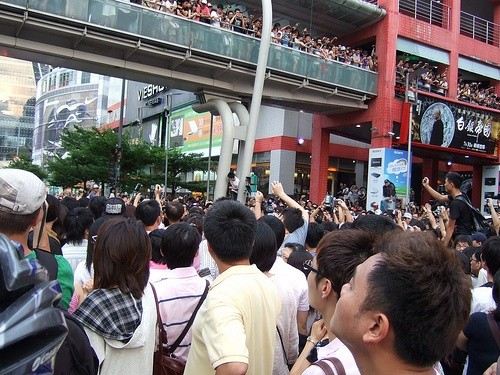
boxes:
[404,90,415,213]
[2,111,21,158]
[163,93,182,200]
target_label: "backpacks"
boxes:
[147,279,210,375]
[451,191,497,237]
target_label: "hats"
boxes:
[191,203,198,206]
[104,198,126,214]
[93,184,99,188]
[472,232,487,242]
[0,168,49,216]
[401,213,413,219]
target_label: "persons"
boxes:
[267,23,377,92]
[457,78,500,109]
[0,169,500,375]
[396,58,448,96]
[88,0,262,64]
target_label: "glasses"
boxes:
[469,257,479,262]
[303,259,336,288]
